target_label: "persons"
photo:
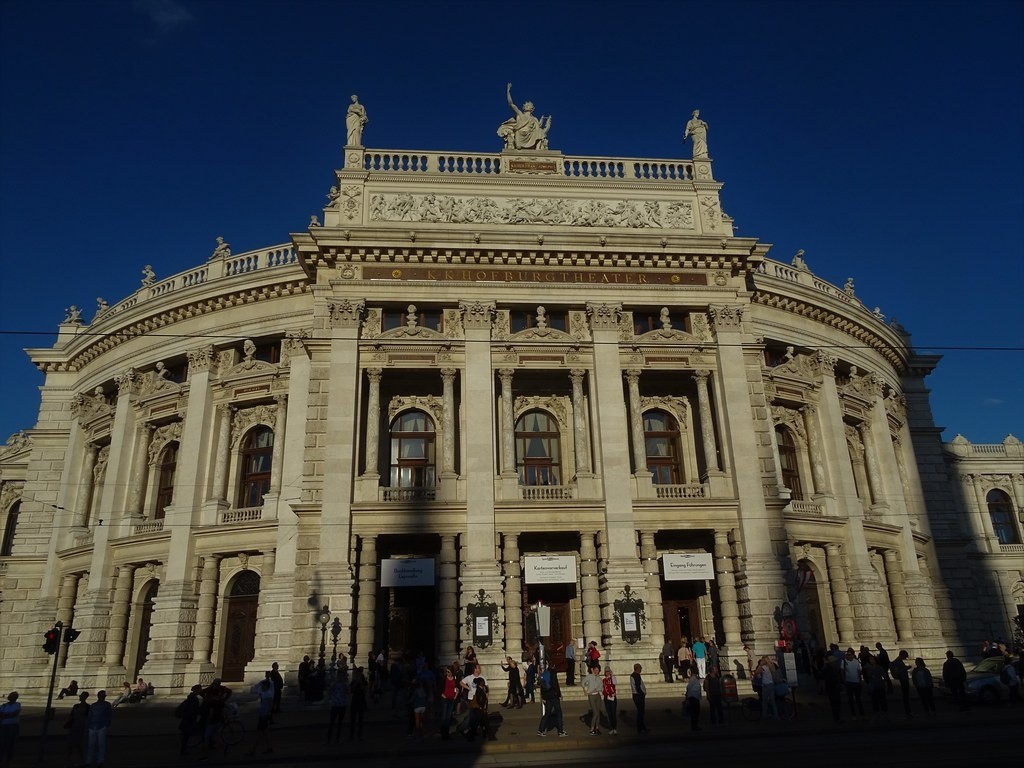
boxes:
[110,678,146,708]
[55,680,78,699]
[178,678,232,762]
[979,637,1010,662]
[682,109,709,159]
[63,690,111,767]
[256,662,284,756]
[297,640,651,743]
[506,82,552,150]
[1,691,22,767]
[345,95,368,145]
[370,192,693,229]
[1000,651,1024,708]
[663,634,967,731]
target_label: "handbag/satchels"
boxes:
[469,694,486,709]
[63,716,74,729]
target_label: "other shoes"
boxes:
[335,739,343,744]
[638,727,652,733]
[691,726,704,731]
[557,730,569,737]
[322,740,331,745]
[244,751,256,757]
[537,730,547,736]
[198,757,208,762]
[833,712,938,724]
[467,736,476,742]
[609,729,618,735]
[589,729,602,735]
[441,735,451,741]
[180,751,192,756]
[261,748,274,754]
[488,736,498,741]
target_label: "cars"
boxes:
[937,654,1024,706]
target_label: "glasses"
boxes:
[446,670,452,673]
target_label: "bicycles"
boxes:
[741,683,797,721]
[180,705,246,747]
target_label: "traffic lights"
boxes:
[42,630,58,654]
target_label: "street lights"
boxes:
[317,605,330,677]
